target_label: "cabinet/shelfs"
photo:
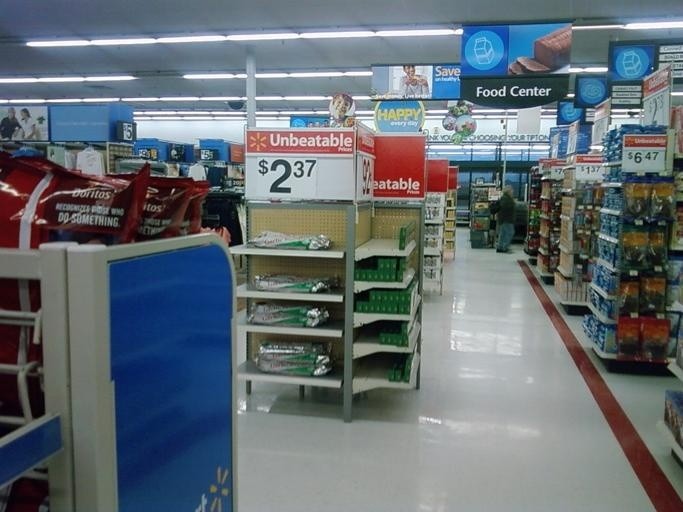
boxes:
[468,157,683,452]
[228,191,456,422]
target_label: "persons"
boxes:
[0,107,40,140]
[496,185,515,254]
[332,95,353,120]
[399,65,428,97]
